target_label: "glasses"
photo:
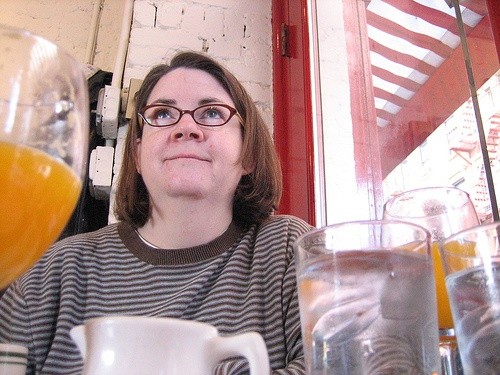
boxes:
[136,104,247,127]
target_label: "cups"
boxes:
[293,219,441,375]
[437,220,500,375]
[0,22,91,302]
[0,343,30,375]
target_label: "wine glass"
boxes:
[377,188,482,375]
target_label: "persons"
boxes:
[0,50,327,375]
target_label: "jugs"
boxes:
[67,316,271,375]
